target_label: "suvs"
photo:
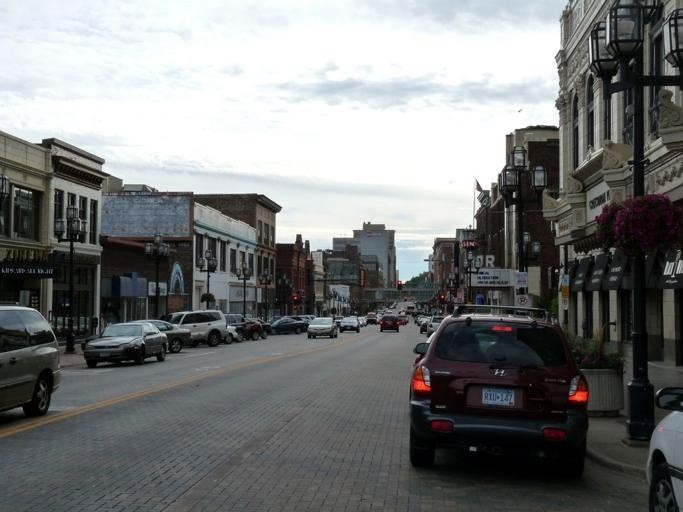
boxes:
[158,308,227,348]
[406,302,594,487]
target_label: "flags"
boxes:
[475,180,483,192]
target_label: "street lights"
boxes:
[257,267,274,322]
[141,229,172,319]
[423,257,446,306]
[234,260,254,318]
[461,248,481,302]
[511,230,543,292]
[195,247,219,310]
[425,269,460,304]
[50,202,89,357]
[583,0,683,448]
[497,141,552,315]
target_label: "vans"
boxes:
[0,303,67,423]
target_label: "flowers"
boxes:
[591,192,679,258]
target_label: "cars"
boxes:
[81,320,169,368]
[639,377,683,512]
[222,295,445,345]
[79,319,192,355]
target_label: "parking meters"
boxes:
[88,317,98,335]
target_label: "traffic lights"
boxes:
[396,280,402,291]
[292,293,299,305]
